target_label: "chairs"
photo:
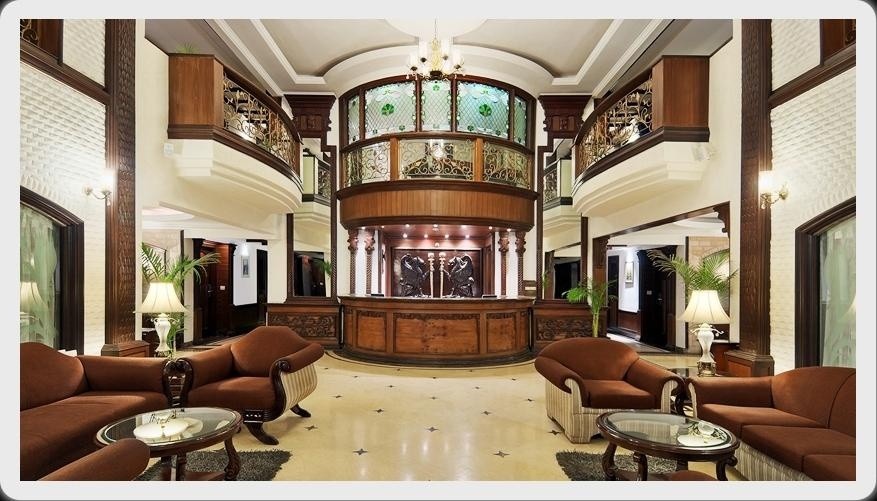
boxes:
[534,337,685,444]
[176,325,323,445]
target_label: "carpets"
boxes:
[555,450,679,481]
[134,449,293,480]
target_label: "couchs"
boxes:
[685,367,856,481]
[36,437,150,480]
[21,342,176,480]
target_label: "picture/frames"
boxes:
[241,256,250,279]
[624,262,634,283]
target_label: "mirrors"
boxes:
[287,131,337,303]
[538,130,587,301]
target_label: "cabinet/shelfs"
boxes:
[697,339,740,375]
[193,306,204,346]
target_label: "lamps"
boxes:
[83,168,115,205]
[406,20,467,82]
[132,282,190,353]
[677,289,730,370]
[757,169,791,210]
